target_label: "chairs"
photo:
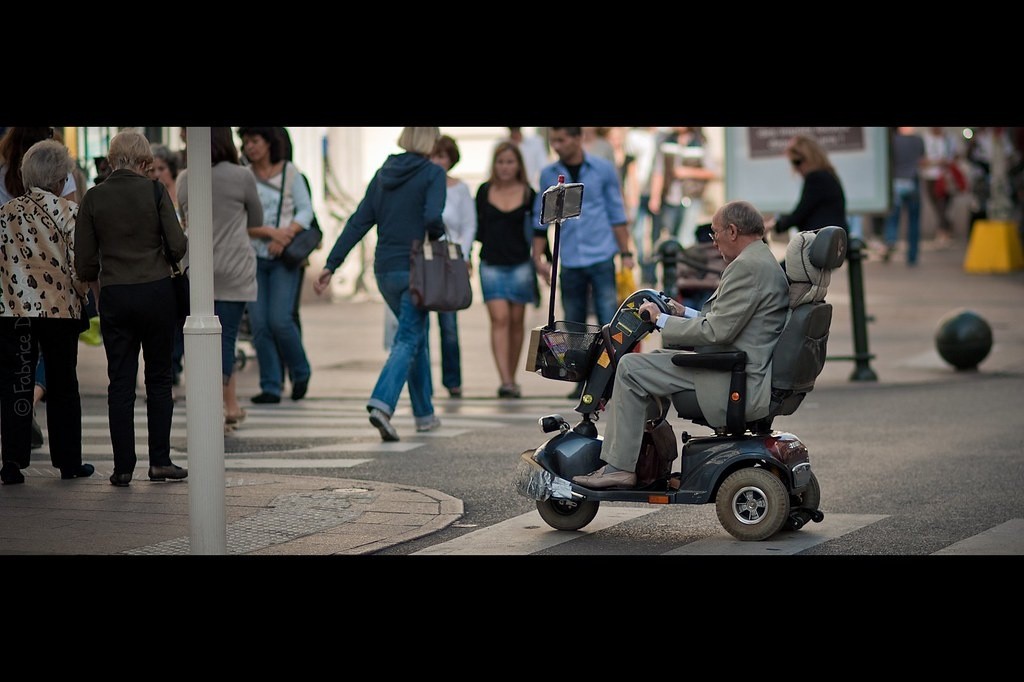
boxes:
[670,226,844,436]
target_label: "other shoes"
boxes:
[369,408,400,441]
[251,391,282,403]
[416,415,439,431]
[224,407,245,427]
[291,374,310,400]
[498,384,521,398]
[449,385,460,395]
[0,466,24,484]
[32,416,44,449]
[61,463,95,480]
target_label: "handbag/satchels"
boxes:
[169,272,190,316]
[273,163,323,264]
[636,417,678,482]
[409,223,473,311]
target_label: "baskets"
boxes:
[538,319,603,380]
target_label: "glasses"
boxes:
[709,225,742,242]
[792,157,806,169]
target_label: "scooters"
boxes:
[512,226,850,541]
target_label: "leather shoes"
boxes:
[149,464,188,481]
[573,465,636,490]
[108,470,132,488]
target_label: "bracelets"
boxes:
[621,253,632,257]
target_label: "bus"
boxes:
[722,127,892,222]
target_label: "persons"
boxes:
[764,136,847,280]
[2,125,322,489]
[598,125,721,276]
[474,143,554,398]
[572,201,789,487]
[877,128,1021,271]
[529,126,638,398]
[420,135,478,398]
[318,127,450,442]
